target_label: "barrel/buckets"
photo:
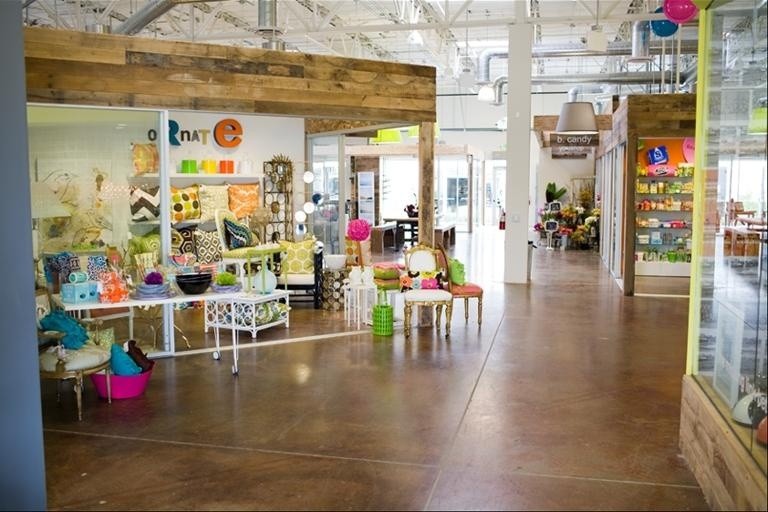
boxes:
[372,304,393,336]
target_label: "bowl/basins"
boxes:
[176,274,212,294]
[90,370,153,399]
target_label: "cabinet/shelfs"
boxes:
[635,139,696,277]
[128,143,263,266]
[350,155,379,226]
[263,154,293,242]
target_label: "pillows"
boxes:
[171,182,264,266]
[226,301,292,325]
[279,239,315,273]
[128,142,162,269]
[344,235,404,289]
[41,308,155,376]
[41,250,129,303]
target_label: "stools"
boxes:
[247,247,287,293]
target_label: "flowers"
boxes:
[533,179,601,250]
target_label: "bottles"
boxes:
[68,271,89,283]
[112,256,122,278]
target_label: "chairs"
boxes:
[314,199,347,254]
[35,286,112,421]
[435,244,483,325]
[273,237,323,306]
[214,210,267,293]
[403,244,454,339]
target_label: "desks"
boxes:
[724,209,768,256]
[344,278,377,330]
[382,217,442,250]
[50,286,240,375]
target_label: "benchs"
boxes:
[429,223,456,249]
[371,225,404,255]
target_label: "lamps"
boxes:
[586,0,608,50]
[747,99,767,136]
[29,181,72,288]
[556,102,599,136]
[458,9,475,88]
[478,9,496,102]
